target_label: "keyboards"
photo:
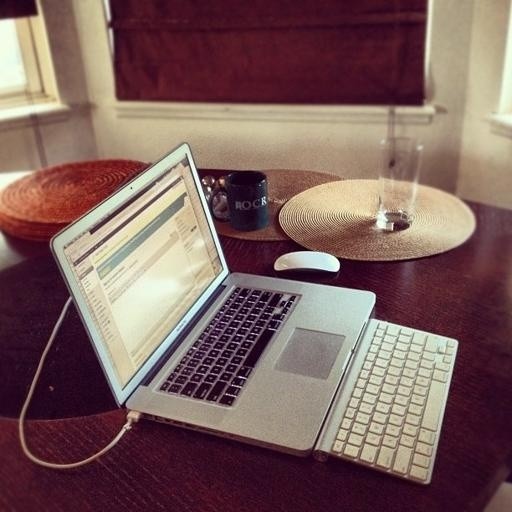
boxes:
[312,319,459,485]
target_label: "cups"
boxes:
[201,173,231,219]
[374,135,425,232]
[207,168,270,234]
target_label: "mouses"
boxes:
[273,250,341,273]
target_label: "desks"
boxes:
[3,168,512,511]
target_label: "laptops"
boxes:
[49,141,378,458]
